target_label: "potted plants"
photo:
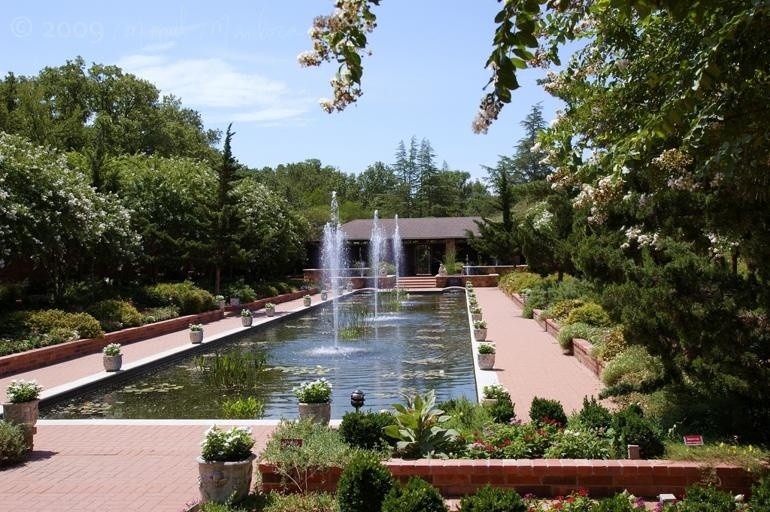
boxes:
[265,303,275,317]
[187,323,204,344]
[465,280,496,370]
[195,423,257,505]
[0,376,45,428]
[303,293,311,306]
[482,384,504,408]
[241,307,252,326]
[336,286,344,295]
[103,343,123,371]
[320,289,327,300]
[346,281,353,292]
[291,378,333,427]
